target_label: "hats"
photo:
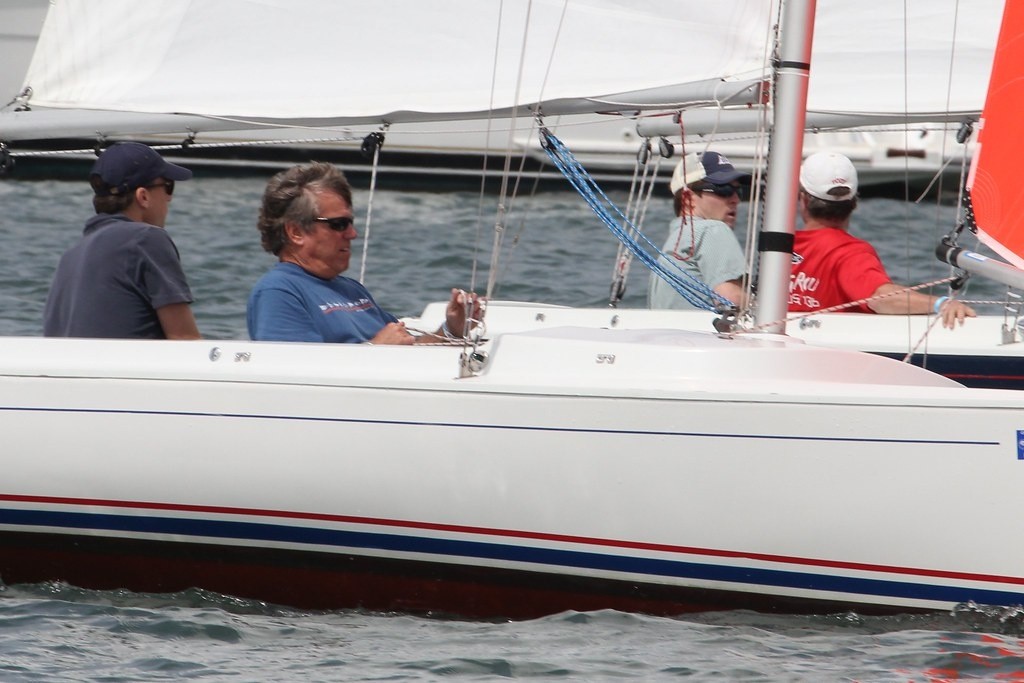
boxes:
[88,141,193,196]
[670,151,750,196]
[800,152,859,201]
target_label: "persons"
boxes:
[44,141,203,343]
[246,161,486,345]
[649,151,978,329]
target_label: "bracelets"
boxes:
[934,296,949,311]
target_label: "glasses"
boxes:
[689,182,743,202]
[137,180,174,196]
[314,216,355,231]
